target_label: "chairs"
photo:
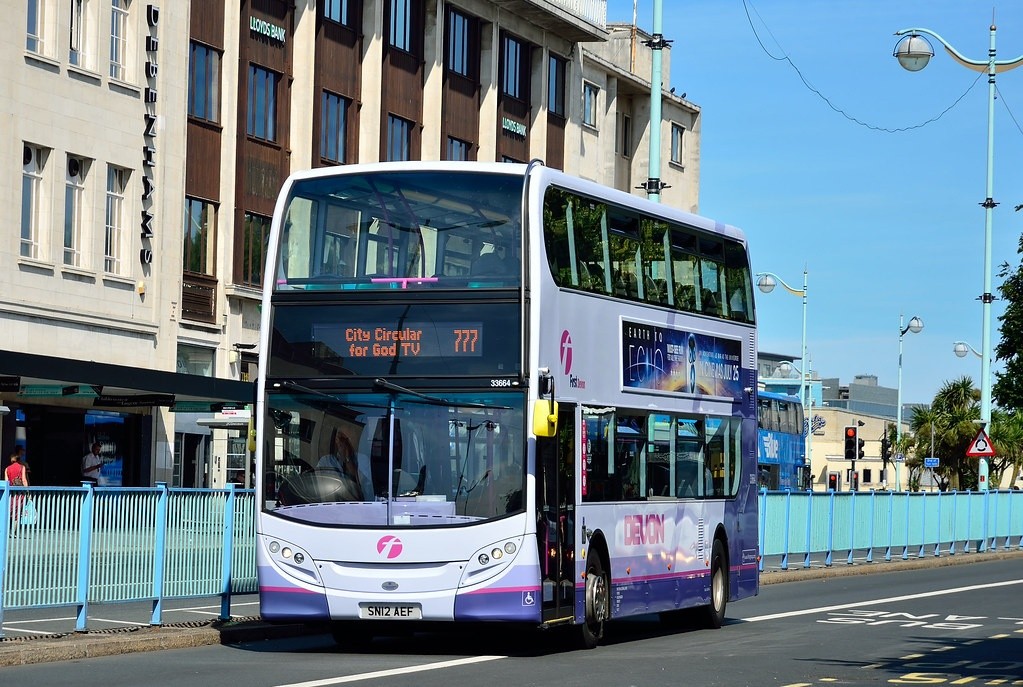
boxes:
[469,253,509,276]
[549,254,732,318]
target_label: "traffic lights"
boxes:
[829,473,838,493]
[844,426,858,461]
[858,438,864,459]
[849,472,858,491]
[881,438,892,462]
[892,425,897,442]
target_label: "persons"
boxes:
[12,444,31,485]
[78,441,105,487]
[4,453,31,539]
[315,424,378,501]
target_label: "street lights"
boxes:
[893,8,1023,492]
[895,315,926,493]
[951,339,992,427]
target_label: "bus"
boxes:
[253,159,761,648]
[754,388,805,493]
